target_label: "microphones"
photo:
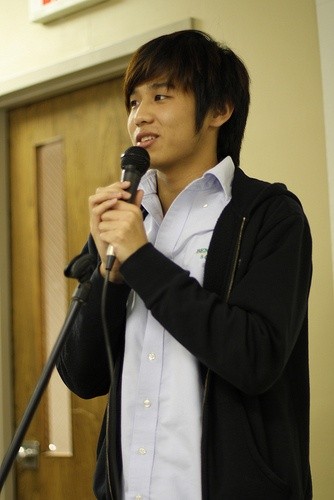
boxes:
[105,146,151,270]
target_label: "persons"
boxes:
[56,29,316,500]
